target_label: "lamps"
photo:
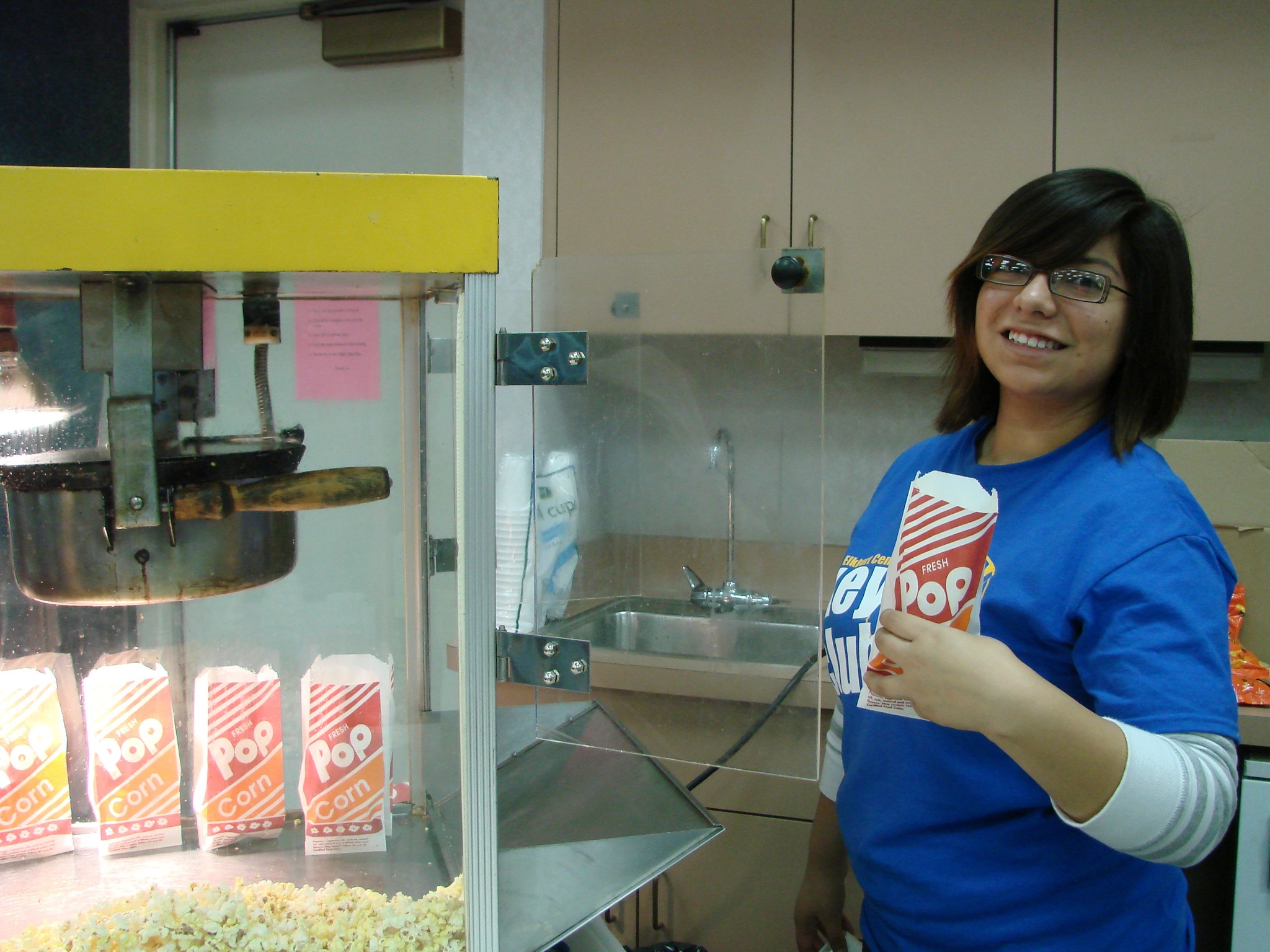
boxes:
[0,298,86,434]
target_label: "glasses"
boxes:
[975,252,1131,304]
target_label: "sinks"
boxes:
[537,594,827,672]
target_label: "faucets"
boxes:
[681,427,771,611]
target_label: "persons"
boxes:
[794,168,1241,952]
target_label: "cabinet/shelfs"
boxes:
[603,807,870,952]
[539,0,1270,341]
